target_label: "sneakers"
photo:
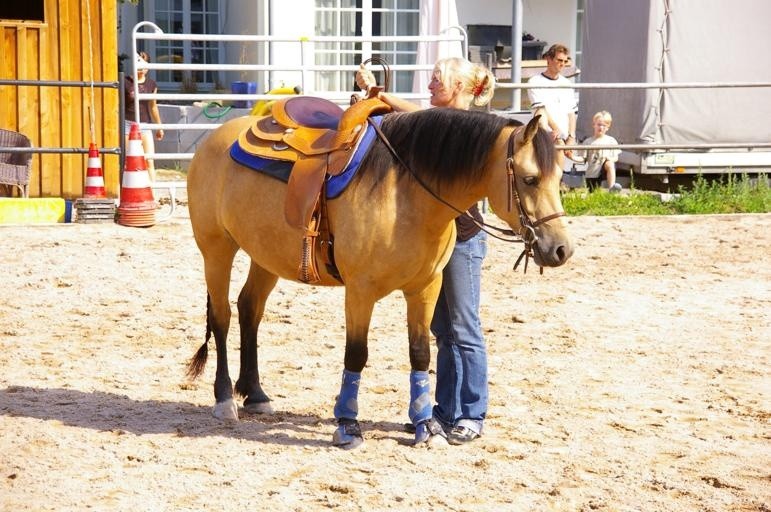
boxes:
[609,183,623,195]
[447,426,479,445]
[404,423,432,435]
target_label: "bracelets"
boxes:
[569,133,578,139]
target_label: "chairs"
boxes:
[1,129,32,201]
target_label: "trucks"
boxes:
[576,1,771,188]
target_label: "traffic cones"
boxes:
[76,142,113,225]
[113,122,162,229]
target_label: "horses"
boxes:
[184,105,573,450]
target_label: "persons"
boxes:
[353,55,499,449]
[581,111,623,196]
[125,52,165,184]
[526,44,582,189]
